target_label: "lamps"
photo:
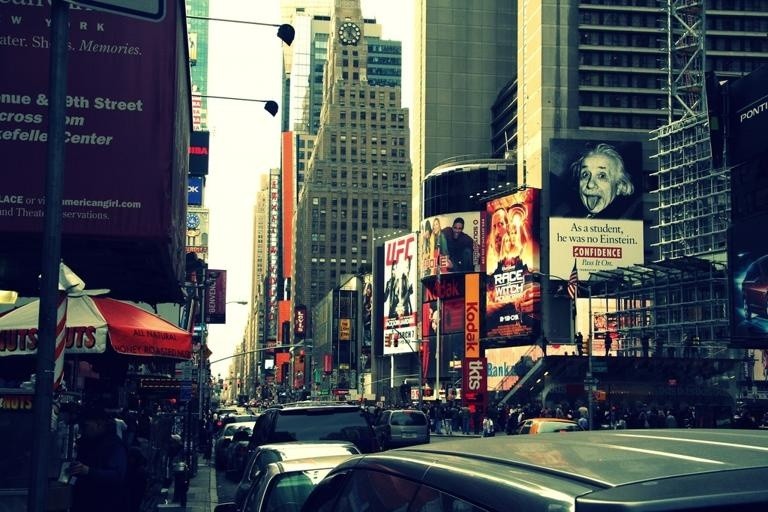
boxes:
[186,16,295,117]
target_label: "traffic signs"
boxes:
[584,377,599,385]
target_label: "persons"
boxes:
[604,333,612,357]
[362,283,372,323]
[423,303,446,377]
[61,398,217,510]
[488,204,529,266]
[574,331,584,355]
[640,330,700,359]
[568,143,637,220]
[384,257,400,320]
[401,255,416,314]
[423,215,478,274]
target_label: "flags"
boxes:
[568,264,577,300]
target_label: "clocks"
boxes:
[186,214,200,229]
[338,22,361,45]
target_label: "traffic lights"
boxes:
[385,333,391,347]
[581,339,588,357]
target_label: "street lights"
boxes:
[532,271,593,431]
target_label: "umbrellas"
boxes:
[0,289,195,511]
